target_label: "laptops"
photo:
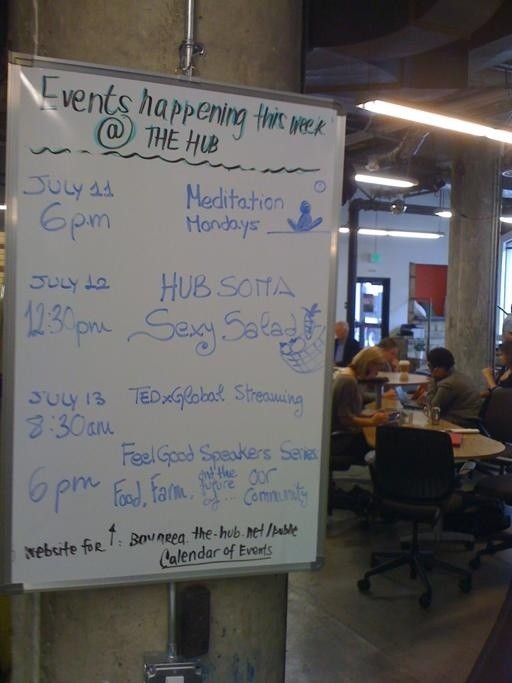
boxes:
[394,385,425,409]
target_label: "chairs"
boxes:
[326,339,512,609]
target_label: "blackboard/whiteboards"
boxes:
[1,50,348,594]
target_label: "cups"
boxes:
[399,360,410,381]
[431,407,441,425]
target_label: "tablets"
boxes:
[388,411,407,421]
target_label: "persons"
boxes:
[500,313,512,344]
[329,345,390,472]
[372,337,402,374]
[335,319,364,367]
[414,346,483,428]
[477,343,512,418]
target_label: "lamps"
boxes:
[356,69,511,146]
[351,160,418,189]
[432,190,512,223]
[338,192,444,240]
